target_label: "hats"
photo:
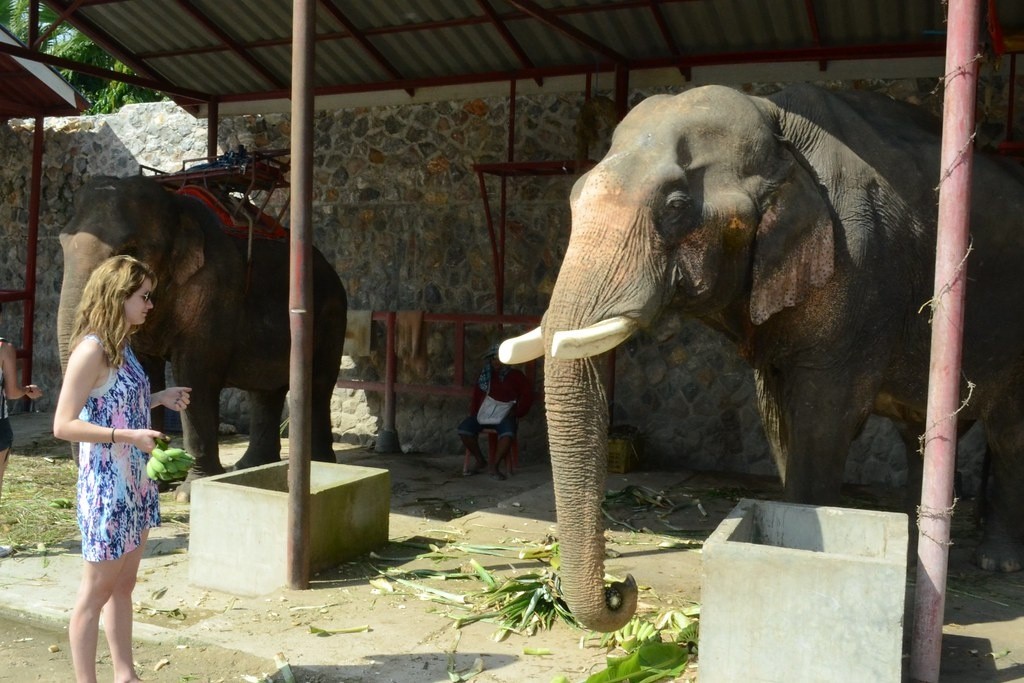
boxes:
[480,343,499,359]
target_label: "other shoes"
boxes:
[0,545,12,556]
[486,469,503,480]
[469,461,487,473]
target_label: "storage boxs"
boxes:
[607,439,645,474]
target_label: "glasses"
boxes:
[133,292,150,301]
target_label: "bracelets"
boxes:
[28,387,33,394]
[111,427,116,443]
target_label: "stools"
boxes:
[462,428,520,476]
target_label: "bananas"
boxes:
[613,615,661,643]
[145,438,197,480]
[675,621,699,644]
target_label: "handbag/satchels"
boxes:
[477,396,517,425]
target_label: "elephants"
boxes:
[498,85,1024,633]
[57,174,348,504]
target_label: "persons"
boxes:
[53,256,192,683]
[0,337,43,499]
